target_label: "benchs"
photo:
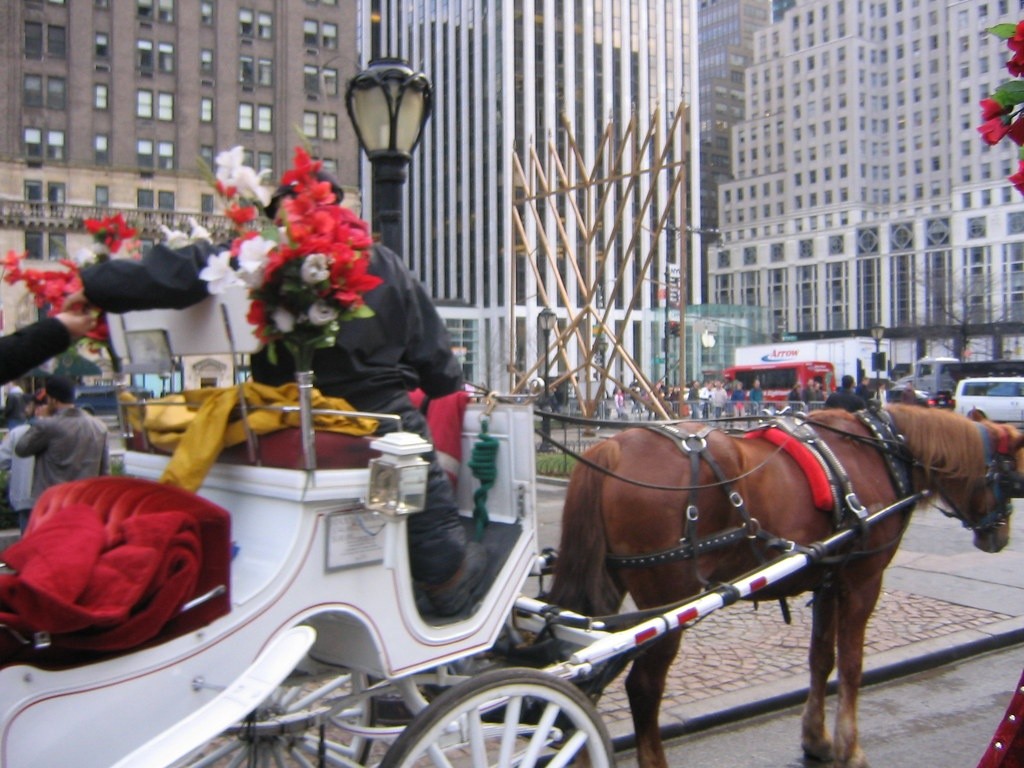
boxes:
[93,282,406,507]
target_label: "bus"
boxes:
[720,361,837,402]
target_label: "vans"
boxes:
[954,376,1024,431]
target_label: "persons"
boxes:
[855,378,870,399]
[900,380,915,406]
[608,378,823,429]
[825,376,867,412]
[251,167,489,618]
[7,385,49,421]
[0,402,47,534]
[16,374,112,501]
[873,382,887,404]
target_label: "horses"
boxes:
[548,401,1024,768]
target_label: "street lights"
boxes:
[871,326,884,401]
[344,57,434,264]
[538,306,556,453]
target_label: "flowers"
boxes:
[0,211,142,355]
[198,142,390,374]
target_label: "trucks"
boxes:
[892,356,1024,400]
[0,244,1024,768]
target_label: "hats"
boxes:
[44,373,77,403]
[262,171,344,220]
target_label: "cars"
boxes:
[74,385,151,415]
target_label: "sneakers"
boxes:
[426,540,486,617]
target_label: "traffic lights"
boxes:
[670,322,680,337]
